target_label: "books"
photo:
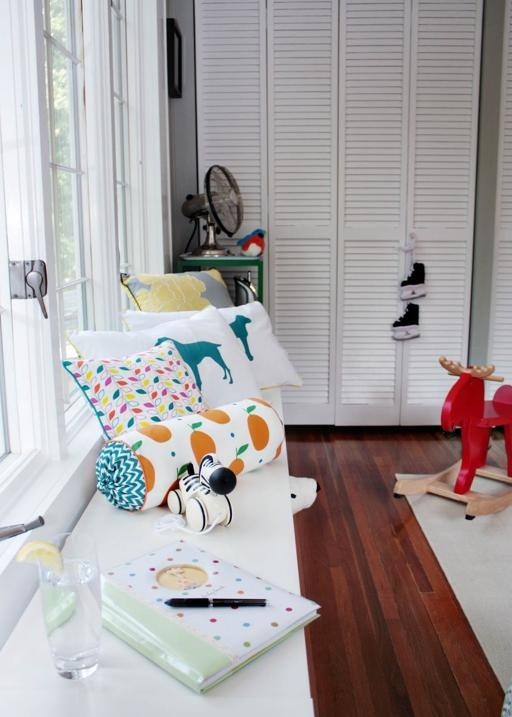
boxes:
[101,536,322,693]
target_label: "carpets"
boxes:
[390,471,512,696]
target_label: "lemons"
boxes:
[16,541,64,572]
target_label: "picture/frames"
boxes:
[165,17,185,100]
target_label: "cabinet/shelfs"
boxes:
[175,256,265,310]
[193,0,488,433]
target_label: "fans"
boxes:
[179,164,243,255]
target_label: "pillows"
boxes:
[94,394,285,512]
[62,339,208,442]
[118,269,232,316]
[67,305,263,411]
[120,300,305,392]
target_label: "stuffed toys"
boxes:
[238,229,266,257]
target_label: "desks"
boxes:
[0,384,317,715]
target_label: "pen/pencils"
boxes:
[165,598,266,606]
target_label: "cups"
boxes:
[37,533,106,679]
